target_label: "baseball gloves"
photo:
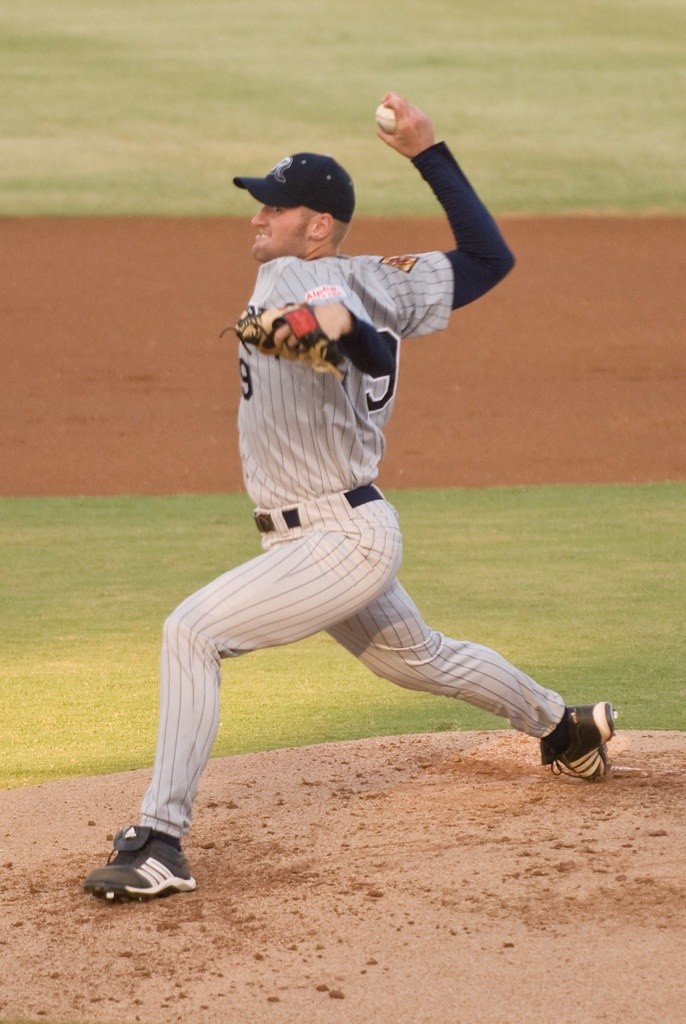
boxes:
[233,299,347,383]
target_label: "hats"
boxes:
[233,153,355,222]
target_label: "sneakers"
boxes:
[540,701,618,782]
[84,825,196,902]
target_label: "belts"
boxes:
[254,485,384,533]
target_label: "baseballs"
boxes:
[374,102,398,134]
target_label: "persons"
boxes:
[80,92,620,901]
